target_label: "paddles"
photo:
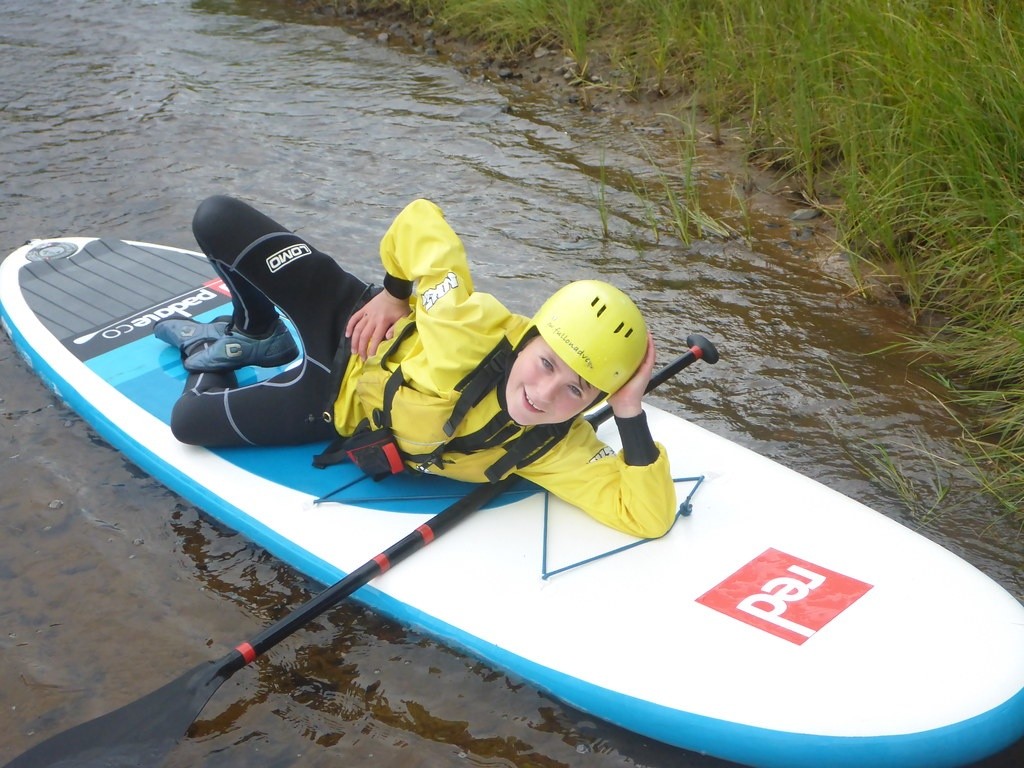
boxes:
[1,332,721,767]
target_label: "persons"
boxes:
[154,194,677,541]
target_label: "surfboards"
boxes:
[0,235,1024,767]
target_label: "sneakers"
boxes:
[184,316,300,372]
[153,315,233,360]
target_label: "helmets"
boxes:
[512,279,649,414]
